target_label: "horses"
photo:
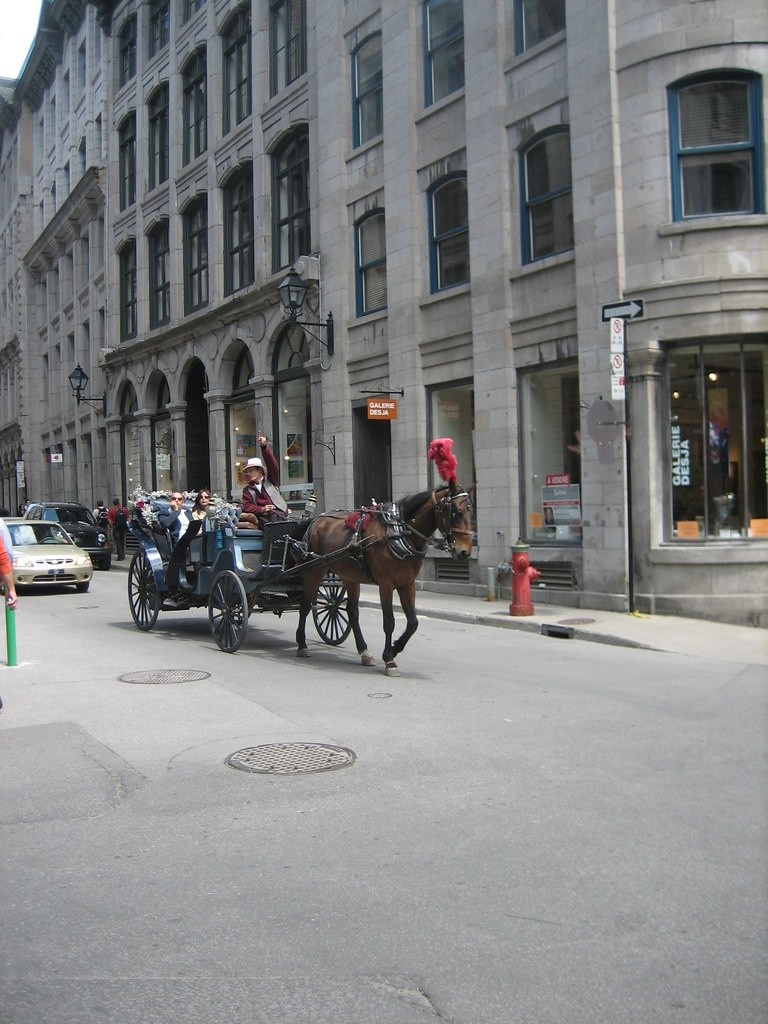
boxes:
[295,476,474,677]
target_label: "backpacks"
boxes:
[113,506,128,531]
[94,507,109,527]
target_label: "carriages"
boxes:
[126,476,475,678]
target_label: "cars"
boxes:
[1,520,94,595]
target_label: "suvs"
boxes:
[23,501,115,571]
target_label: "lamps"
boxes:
[279,268,335,357]
[154,426,175,454]
[68,362,106,420]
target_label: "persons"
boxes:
[192,489,215,536]
[92,499,110,551]
[24,500,32,510]
[157,492,193,549]
[0,518,20,710]
[108,498,129,561]
[17,504,26,517]
[243,435,288,528]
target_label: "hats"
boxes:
[242,458,267,475]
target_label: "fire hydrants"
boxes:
[509,537,542,616]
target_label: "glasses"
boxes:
[172,497,183,502]
[199,496,209,499]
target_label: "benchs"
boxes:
[136,498,237,557]
[233,527,265,552]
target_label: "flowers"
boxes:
[128,485,241,526]
